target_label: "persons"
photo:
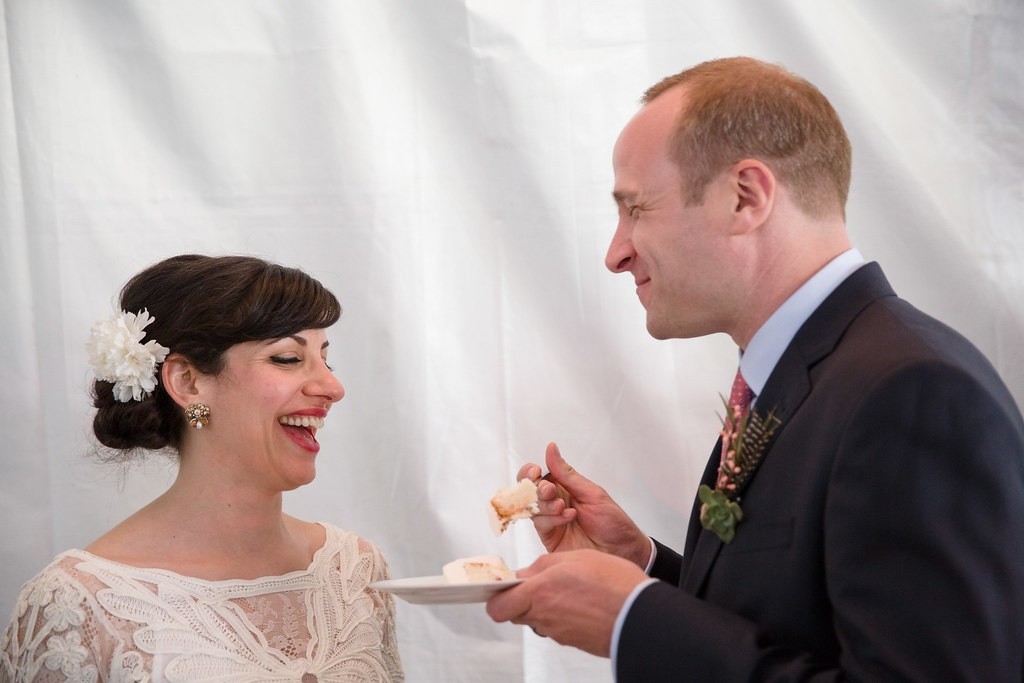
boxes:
[0,254,405,683]
[486,55,1024,683]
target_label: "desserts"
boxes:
[486,476,541,535]
[441,553,517,585]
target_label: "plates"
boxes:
[369,576,524,605]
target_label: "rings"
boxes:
[532,626,547,637]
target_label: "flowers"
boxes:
[697,392,759,544]
[83,307,173,403]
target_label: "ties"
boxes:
[715,367,756,496]
